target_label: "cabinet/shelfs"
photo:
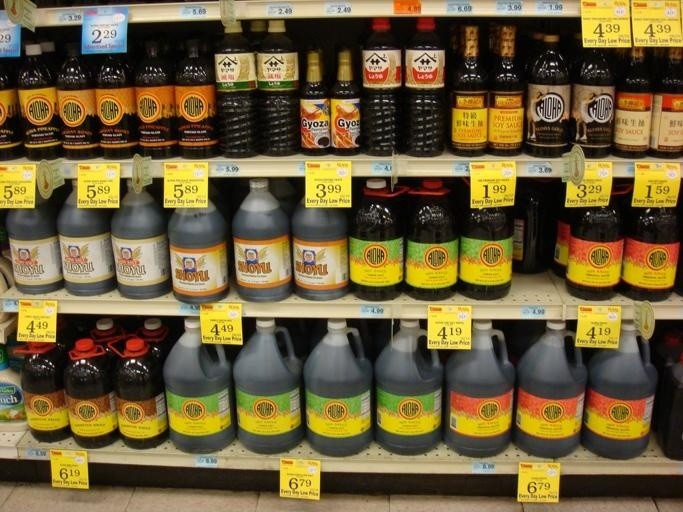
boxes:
[0,0,683,498]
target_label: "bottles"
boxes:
[0,163,681,303]
[0,17,683,163]
[650,326,683,460]
[1,318,180,448]
